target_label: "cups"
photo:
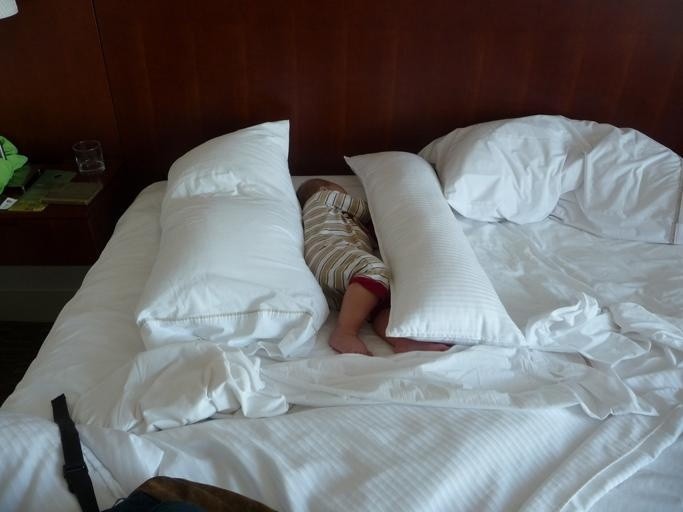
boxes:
[71,139,107,177]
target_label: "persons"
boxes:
[293,179,450,357]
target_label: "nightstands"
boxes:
[1,158,112,321]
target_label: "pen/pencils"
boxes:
[21,169,40,190]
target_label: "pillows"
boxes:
[343,151,527,345]
[133,120,330,357]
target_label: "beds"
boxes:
[0,176,683,512]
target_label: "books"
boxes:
[41,179,103,206]
